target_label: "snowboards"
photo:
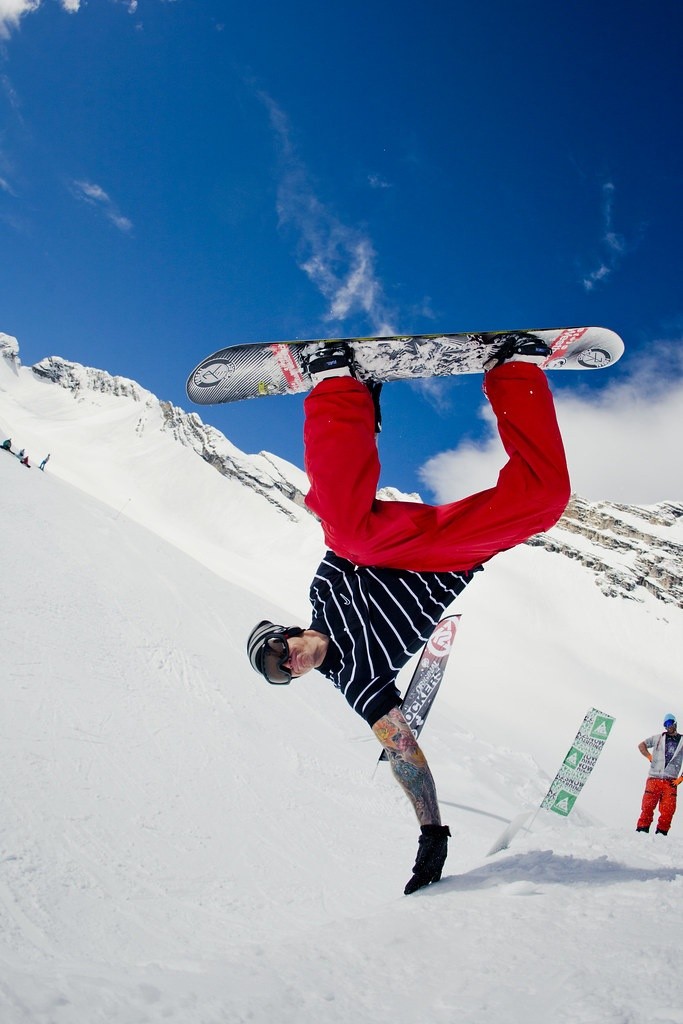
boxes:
[187,325,627,407]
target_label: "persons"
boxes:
[636,714,683,835]
[247,340,571,894]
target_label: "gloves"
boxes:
[671,775,683,786]
[648,756,653,762]
[403,821,452,894]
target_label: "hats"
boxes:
[246,618,289,675]
[664,713,676,722]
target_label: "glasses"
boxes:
[664,719,674,727]
[261,634,292,685]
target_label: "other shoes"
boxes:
[484,332,553,359]
[308,343,352,376]
